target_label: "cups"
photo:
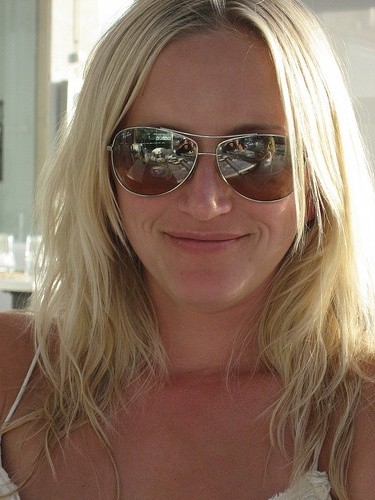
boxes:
[25,234,46,280]
[0,232,16,272]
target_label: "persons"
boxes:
[0,0,375,500]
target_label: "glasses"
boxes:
[106,126,307,203]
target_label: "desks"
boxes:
[0,272,61,310]
